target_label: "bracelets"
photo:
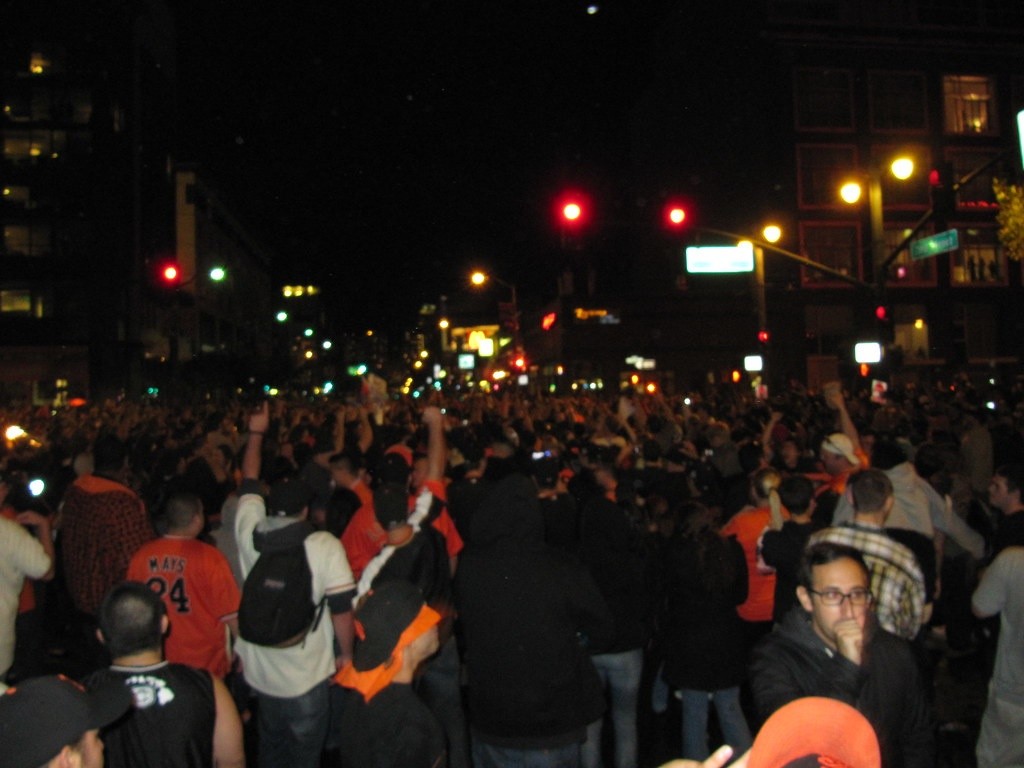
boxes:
[249,429,265,435]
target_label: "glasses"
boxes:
[811,590,872,607]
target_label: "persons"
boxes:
[234,400,351,768]
[963,249,998,282]
[739,543,912,768]
[349,582,442,767]
[0,343,1024,768]
[0,676,131,767]
[970,544,1023,766]
[85,580,243,767]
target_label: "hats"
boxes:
[820,434,860,466]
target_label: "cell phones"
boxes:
[532,451,552,460]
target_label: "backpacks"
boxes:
[238,543,327,647]
